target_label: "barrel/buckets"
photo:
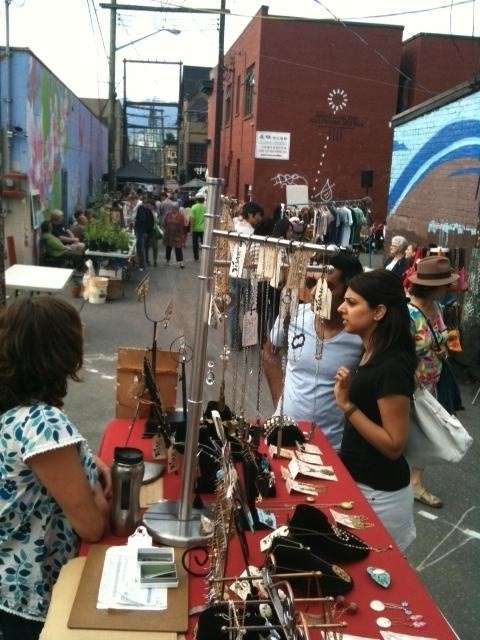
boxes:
[88,276,109,305]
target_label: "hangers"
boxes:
[285,199,367,213]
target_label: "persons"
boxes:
[261,256,366,455]
[405,255,459,508]
[229,201,294,355]
[384,235,411,275]
[0,295,113,640]
[41,181,209,272]
[404,242,418,258]
[332,268,416,554]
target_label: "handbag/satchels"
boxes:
[404,386,473,466]
[438,361,462,412]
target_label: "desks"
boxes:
[2,263,73,298]
[73,418,465,640]
[84,230,140,300]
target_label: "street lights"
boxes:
[109,25,182,197]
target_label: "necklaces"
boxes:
[292,524,379,553]
[267,537,312,556]
[212,235,332,451]
[303,464,335,475]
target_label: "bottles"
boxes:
[109,446,145,537]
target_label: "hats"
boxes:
[408,256,459,286]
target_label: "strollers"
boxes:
[362,223,384,256]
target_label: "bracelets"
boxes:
[345,405,358,418]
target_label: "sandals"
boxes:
[414,487,442,507]
